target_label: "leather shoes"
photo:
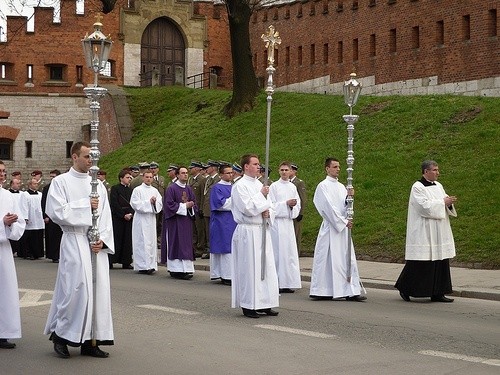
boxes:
[400,291,410,301]
[52,335,69,357]
[81,345,109,357]
[431,296,454,302]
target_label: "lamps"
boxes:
[81,12,114,88]
[343,66,362,115]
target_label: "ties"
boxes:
[156,177,157,181]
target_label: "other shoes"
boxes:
[0,340,16,348]
[279,288,295,293]
[53,259,59,263]
[139,269,155,273]
[123,265,133,269]
[201,254,208,259]
[173,273,192,279]
[222,279,229,284]
[257,309,279,315]
[109,264,113,269]
[346,295,367,300]
[31,255,35,260]
[243,309,259,317]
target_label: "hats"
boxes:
[97,171,106,175]
[290,164,299,170]
[30,170,42,175]
[12,172,20,175]
[128,163,158,173]
[167,160,272,175]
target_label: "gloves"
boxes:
[296,215,303,222]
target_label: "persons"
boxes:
[269,160,302,293]
[309,157,368,302]
[129,170,163,274]
[2,161,307,270]
[230,155,280,318]
[208,164,234,286]
[43,140,115,359]
[394,159,457,303]
[160,166,199,280]
[0,161,27,350]
